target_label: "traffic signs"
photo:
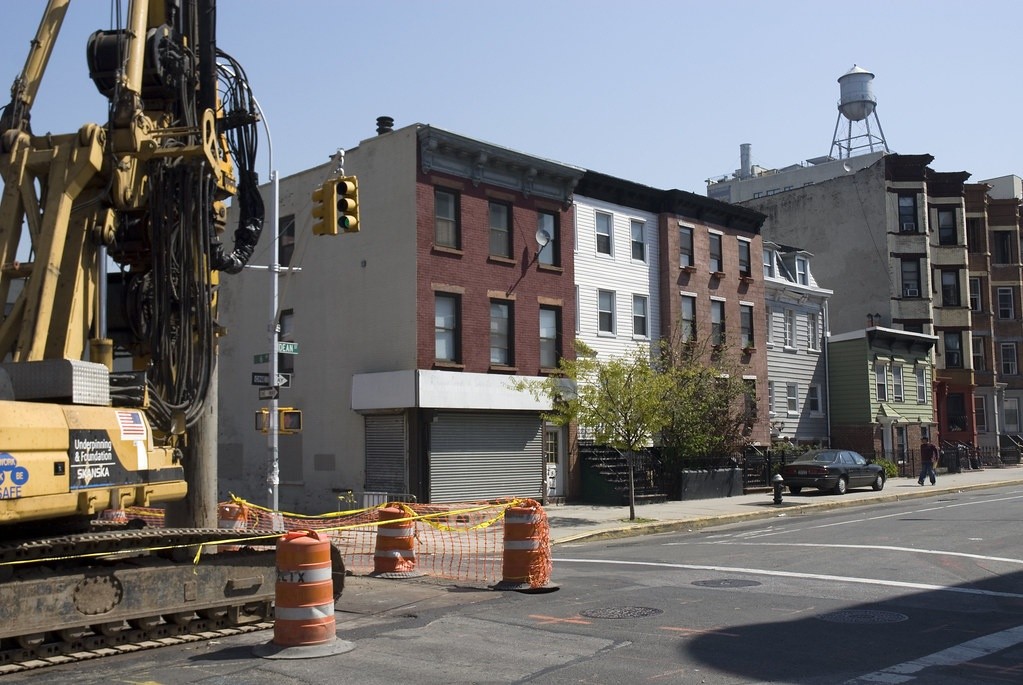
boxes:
[258,386,279,399]
[251,372,291,389]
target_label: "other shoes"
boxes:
[918,481,924,486]
[932,482,935,485]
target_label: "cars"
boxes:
[782,448,887,496]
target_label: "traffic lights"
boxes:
[337,175,360,234]
[279,409,302,433]
[254,406,268,434]
[311,179,339,240]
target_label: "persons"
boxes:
[918,437,939,486]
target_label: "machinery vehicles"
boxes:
[0,0,347,675]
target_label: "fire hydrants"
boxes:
[771,475,784,505]
